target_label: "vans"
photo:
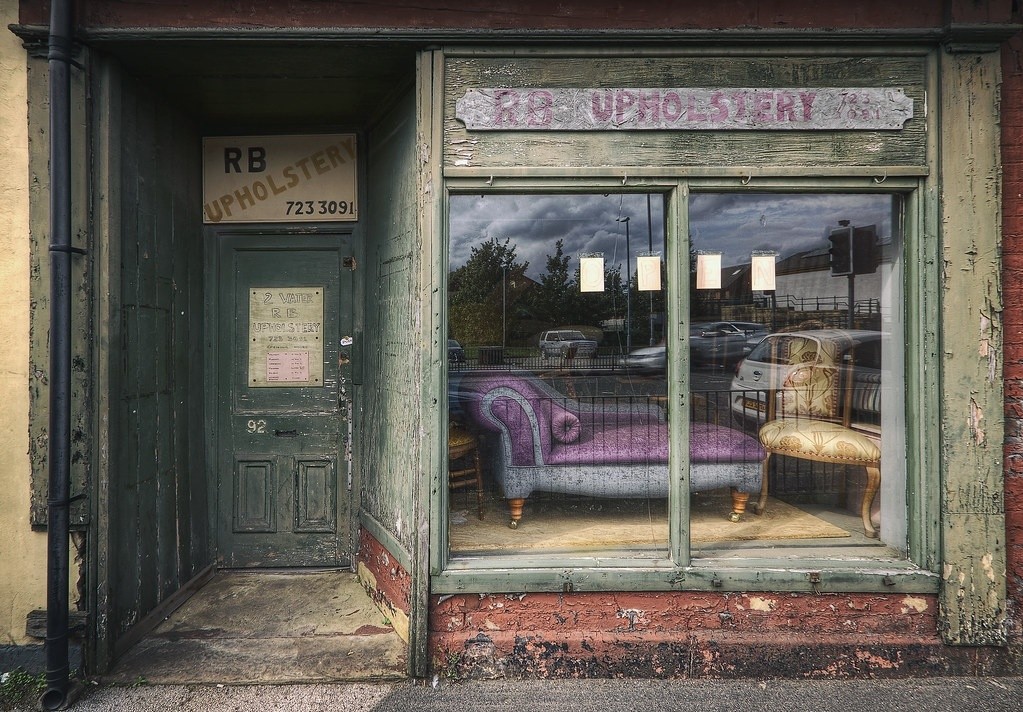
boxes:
[729,328,882,441]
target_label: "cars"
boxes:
[448,339,466,364]
[625,340,667,373]
[688,320,767,363]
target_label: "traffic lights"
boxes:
[829,227,853,277]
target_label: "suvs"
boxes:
[538,330,596,360]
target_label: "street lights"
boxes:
[502,263,509,346]
[621,216,631,354]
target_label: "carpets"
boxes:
[448,488,853,551]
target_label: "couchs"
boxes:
[459,364,769,529]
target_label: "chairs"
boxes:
[755,319,881,538]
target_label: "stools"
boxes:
[449,423,486,521]
